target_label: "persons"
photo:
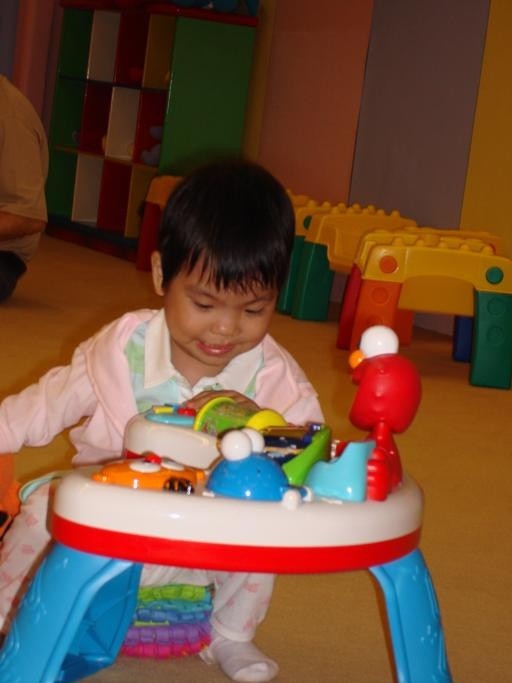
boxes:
[0,73,51,304]
[0,153,324,682]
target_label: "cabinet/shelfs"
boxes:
[46,0,257,258]
